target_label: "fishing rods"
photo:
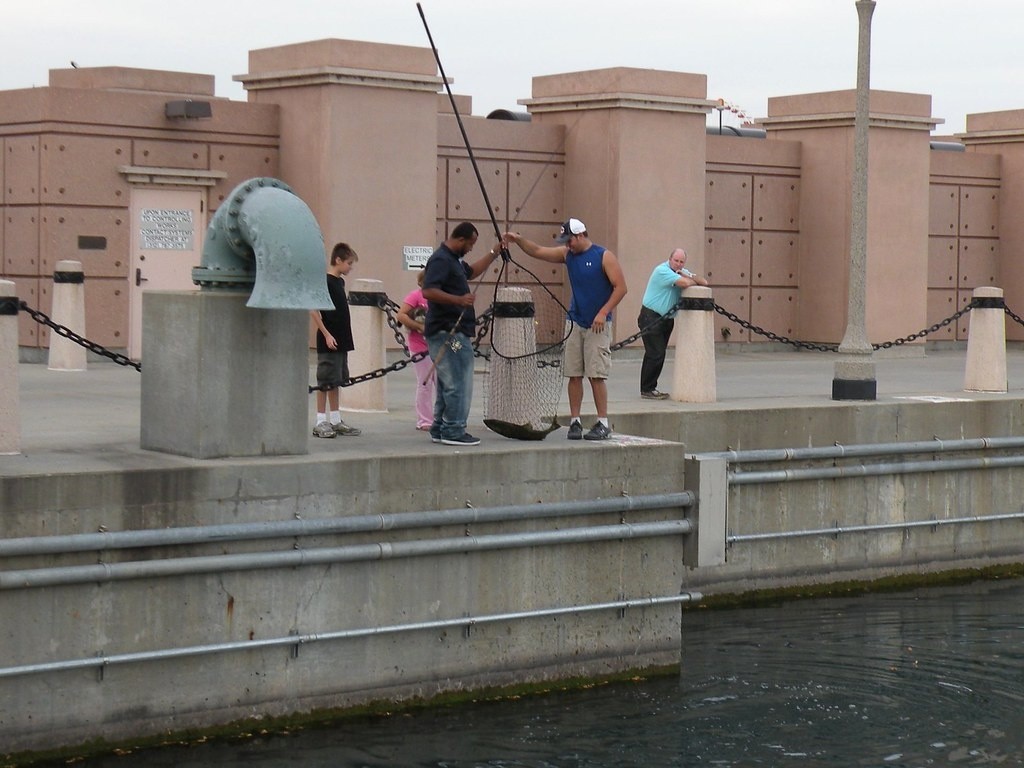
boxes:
[421,67,640,388]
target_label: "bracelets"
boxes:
[490,250,498,258]
[599,309,608,316]
[689,275,693,279]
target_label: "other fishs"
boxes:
[482,412,562,441]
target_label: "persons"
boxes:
[309,242,361,438]
[637,248,708,400]
[397,221,501,445]
[501,218,627,439]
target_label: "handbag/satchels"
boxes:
[406,306,426,334]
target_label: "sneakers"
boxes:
[313,421,337,438]
[429,432,440,442]
[567,419,583,440]
[441,433,480,445]
[583,420,612,440]
[641,389,669,400]
[330,420,361,436]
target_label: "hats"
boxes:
[555,217,587,243]
